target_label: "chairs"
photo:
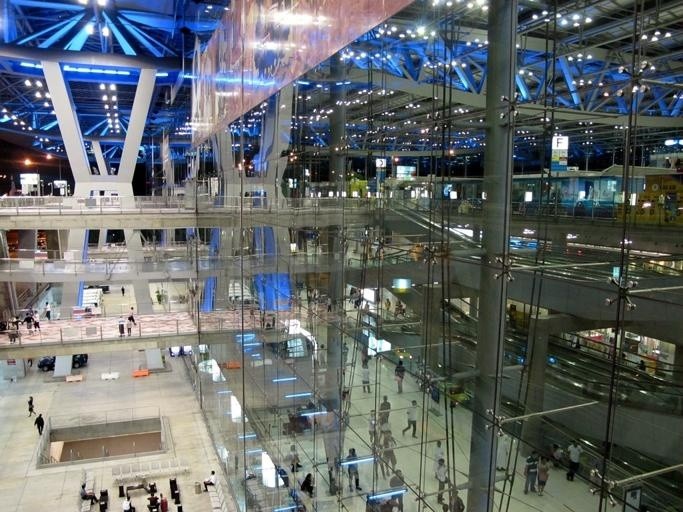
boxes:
[109,457,188,487]
[77,466,97,512]
[201,477,229,512]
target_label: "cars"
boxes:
[36,353,87,372]
[430,379,470,407]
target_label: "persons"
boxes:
[34,414,44,435]
[639,360,646,377]
[80,483,99,505]
[203,471,216,492]
[566,440,584,481]
[523,450,540,494]
[160,493,167,509]
[123,496,135,512]
[7,305,41,345]
[537,456,551,496]
[118,307,137,337]
[608,338,616,361]
[28,397,36,417]
[147,492,159,512]
[43,302,51,322]
[549,443,562,469]
